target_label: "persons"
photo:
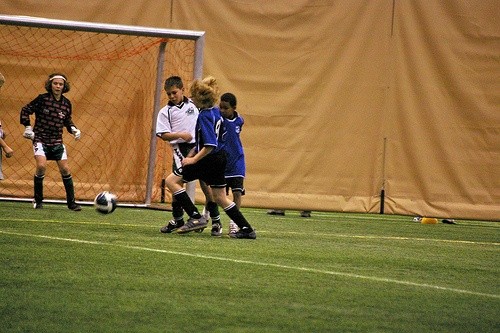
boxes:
[156,76,223,237]
[20,72,82,211]
[165,76,257,239]
[267,209,311,217]
[194,93,245,238]
[0,72,14,180]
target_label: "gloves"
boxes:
[70,125,80,139]
[23,125,34,141]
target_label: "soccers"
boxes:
[94,191,117,214]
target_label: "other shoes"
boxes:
[32,200,43,209]
[68,202,82,211]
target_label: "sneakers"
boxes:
[228,228,256,239]
[160,217,184,232]
[194,219,209,233]
[229,220,240,234]
[211,222,223,236]
[177,214,207,233]
